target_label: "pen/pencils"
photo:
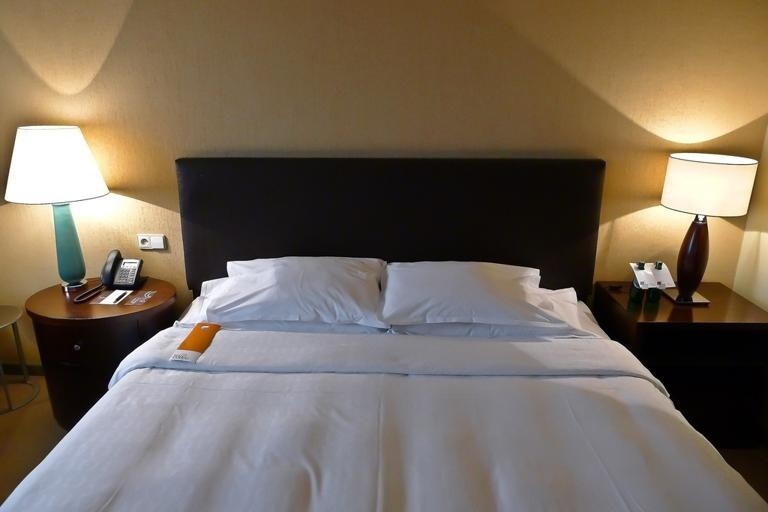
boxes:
[113,292,126,303]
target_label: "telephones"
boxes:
[100,250,143,289]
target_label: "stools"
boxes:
[1,304,41,414]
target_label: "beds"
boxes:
[0,157,767,512]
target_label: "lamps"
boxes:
[4,125,109,292]
[659,152,759,305]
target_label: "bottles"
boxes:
[626,300,660,323]
[630,261,645,301]
[646,261,662,301]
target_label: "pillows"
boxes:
[223,323,381,335]
[205,257,387,331]
[381,261,558,326]
[387,286,603,340]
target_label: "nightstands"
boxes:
[25,278,176,430]
[593,281,768,448]
